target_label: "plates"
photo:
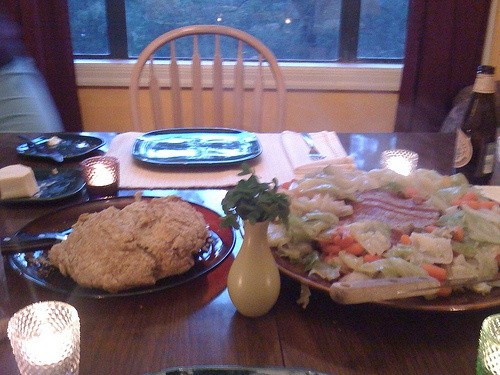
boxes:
[2,162,87,204]
[240,176,500,311]
[15,132,105,159]
[131,127,262,165]
[7,196,235,298]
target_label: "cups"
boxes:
[476,312,500,375]
[80,156,120,200]
[9,300,80,374]
[379,147,419,175]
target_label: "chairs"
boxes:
[130,24,286,133]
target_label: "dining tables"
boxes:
[0,132,500,375]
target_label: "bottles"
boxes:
[453,66,500,187]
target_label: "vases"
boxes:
[220,215,290,317]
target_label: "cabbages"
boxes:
[275,165,500,309]
